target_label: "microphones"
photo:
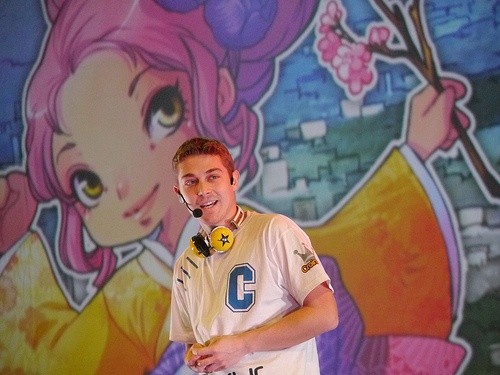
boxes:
[178,190,203,218]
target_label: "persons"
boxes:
[168,138,338,375]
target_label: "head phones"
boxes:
[191,205,245,258]
